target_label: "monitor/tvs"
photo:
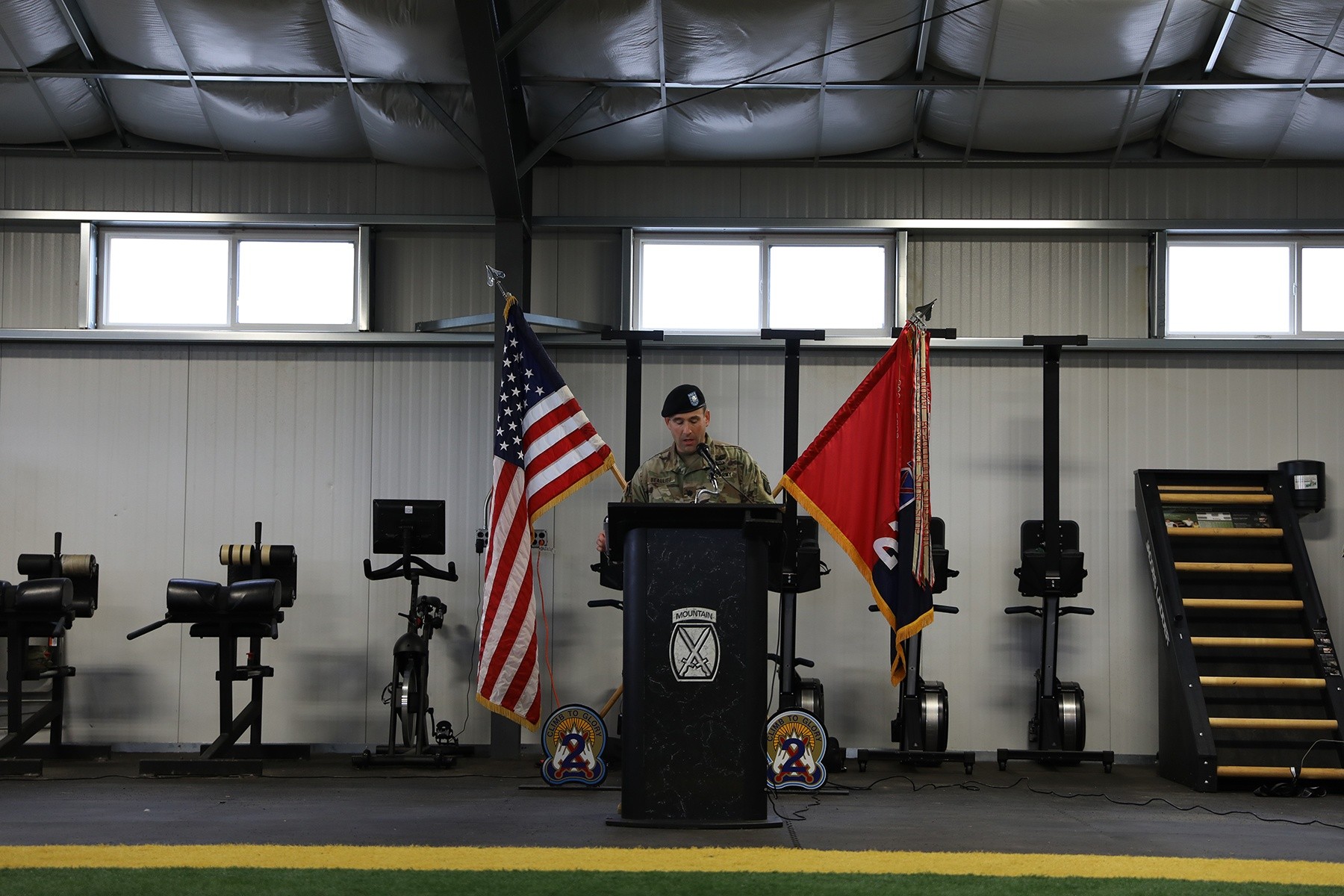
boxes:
[371,498,446,555]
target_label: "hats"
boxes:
[661,384,705,417]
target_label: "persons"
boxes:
[597,384,775,550]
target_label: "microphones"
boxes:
[697,443,722,477]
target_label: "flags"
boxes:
[475,294,615,732]
[779,320,935,687]
